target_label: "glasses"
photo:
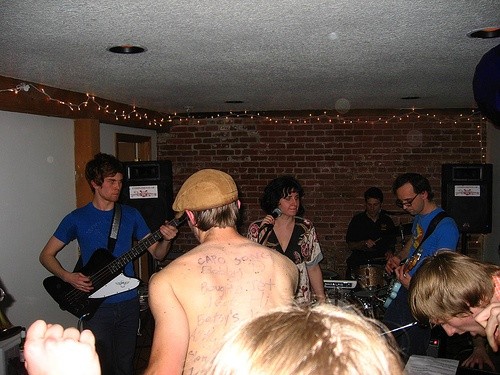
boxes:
[394,194,418,207]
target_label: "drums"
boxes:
[348,260,386,298]
[309,270,339,293]
[348,297,382,319]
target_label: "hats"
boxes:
[172,168,238,211]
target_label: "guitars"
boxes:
[42,212,189,320]
[384,252,421,307]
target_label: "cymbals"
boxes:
[390,222,413,236]
[384,209,409,215]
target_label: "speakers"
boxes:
[440,163,492,234]
[117,161,176,242]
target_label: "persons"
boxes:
[24,320,101,375]
[144,169,298,375]
[39,153,178,375]
[247,175,326,305]
[384,172,459,365]
[346,187,397,304]
[408,248,500,371]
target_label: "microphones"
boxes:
[258,208,281,233]
[379,209,404,215]
